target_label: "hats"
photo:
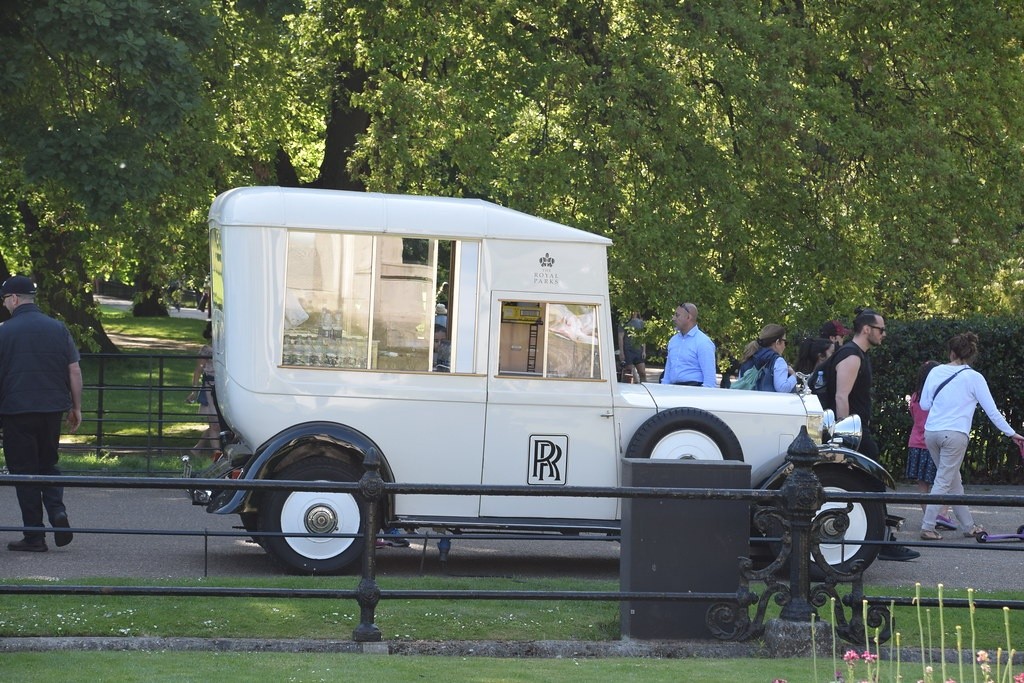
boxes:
[1,276,37,294]
[821,320,853,336]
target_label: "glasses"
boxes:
[782,339,788,346]
[680,303,689,313]
[870,325,886,334]
[1,294,20,301]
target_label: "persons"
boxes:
[793,336,835,379]
[431,314,448,366]
[810,306,922,561]
[737,323,797,394]
[0,275,83,551]
[820,318,853,352]
[187,322,221,459]
[661,302,716,388]
[906,360,959,528]
[917,331,1024,539]
[617,309,647,385]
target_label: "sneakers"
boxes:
[8,535,47,552]
[936,515,958,530]
[879,544,921,560]
[52,511,73,547]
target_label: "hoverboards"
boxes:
[975,439,1024,544]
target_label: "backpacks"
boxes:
[807,347,857,409]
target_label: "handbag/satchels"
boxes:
[731,362,769,390]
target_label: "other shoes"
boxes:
[920,529,943,540]
[964,524,986,537]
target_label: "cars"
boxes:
[185,185,907,580]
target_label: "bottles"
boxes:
[815,371,825,389]
[284,307,357,368]
